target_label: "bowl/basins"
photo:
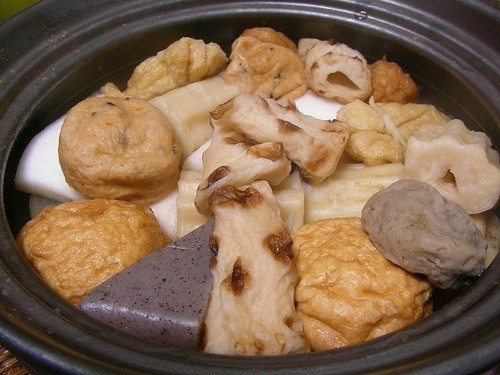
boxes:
[0,0,499,375]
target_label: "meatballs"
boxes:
[241,26,421,105]
[359,178,489,288]
